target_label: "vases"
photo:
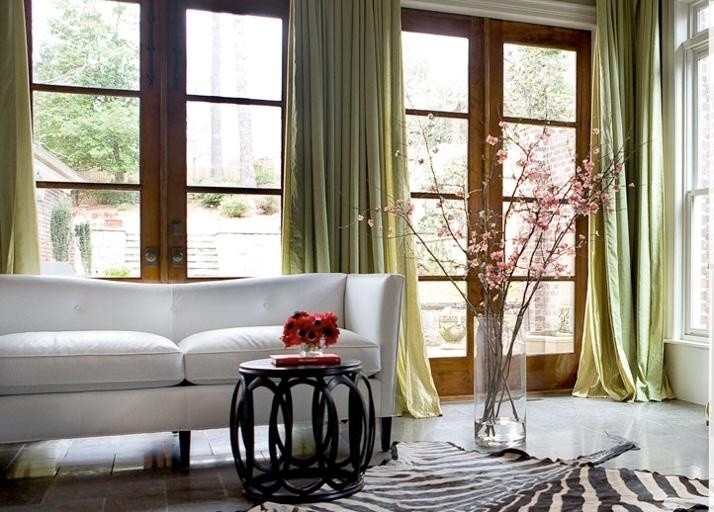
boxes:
[471,314,529,452]
[301,346,323,356]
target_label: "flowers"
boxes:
[332,91,655,439]
[279,309,341,349]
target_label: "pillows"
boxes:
[177,325,382,385]
[2,331,183,395]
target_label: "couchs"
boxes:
[1,271,403,474]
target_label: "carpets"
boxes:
[243,438,710,512]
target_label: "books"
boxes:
[269,353,341,366]
